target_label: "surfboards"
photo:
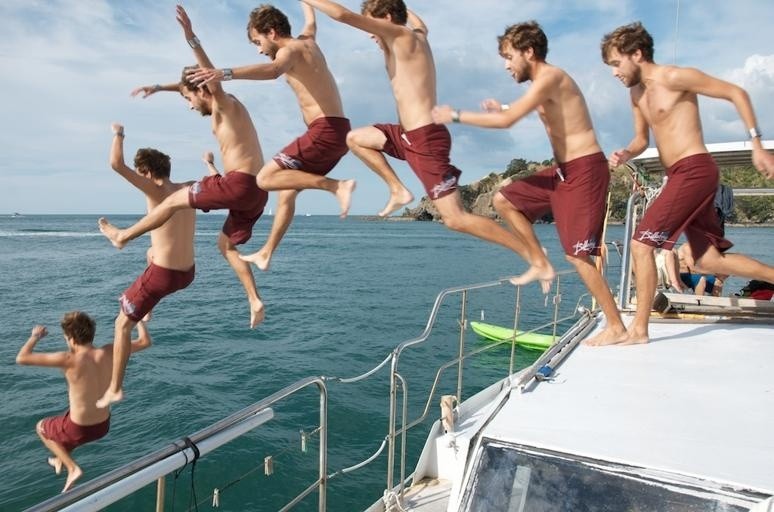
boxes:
[470,321,562,352]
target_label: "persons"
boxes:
[431,20,629,347]
[602,22,773,346]
[665,241,717,296]
[185,0,355,273]
[94,123,221,409]
[16,309,151,494]
[303,0,555,294]
[98,4,269,329]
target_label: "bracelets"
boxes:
[113,131,124,138]
[501,105,510,112]
[32,333,41,339]
[223,67,233,81]
[747,126,763,140]
[205,161,214,165]
[154,83,162,91]
[186,35,201,49]
[453,109,460,121]
